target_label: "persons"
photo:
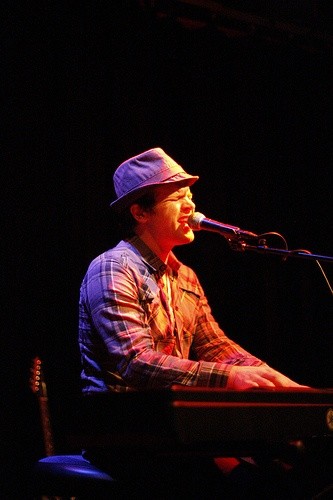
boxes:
[78,148,314,500]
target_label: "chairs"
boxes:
[39,357,82,458]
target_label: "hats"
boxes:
[110,147,200,209]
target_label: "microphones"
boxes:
[188,212,258,239]
